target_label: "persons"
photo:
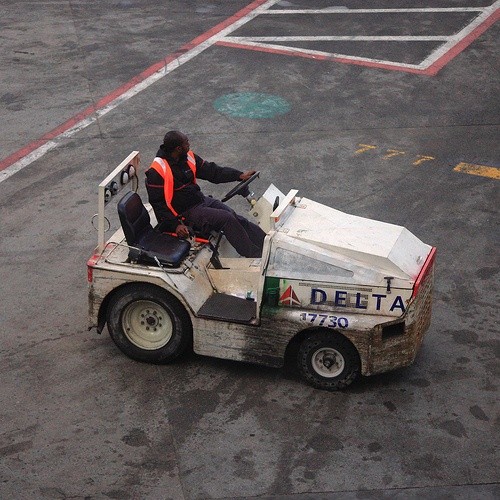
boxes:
[145,130,267,258]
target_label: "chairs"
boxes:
[117,190,191,268]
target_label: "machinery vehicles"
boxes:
[86,150,438,392]
